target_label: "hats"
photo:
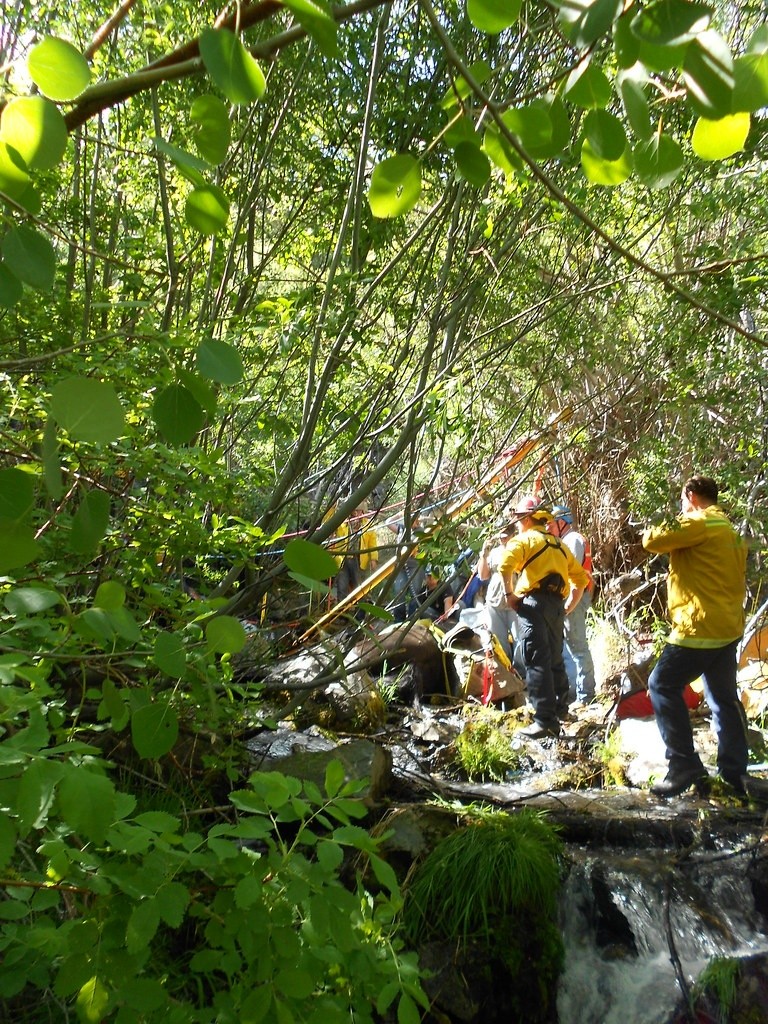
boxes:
[426,566,439,575]
[495,519,515,539]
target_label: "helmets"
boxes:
[550,505,574,524]
[513,495,547,515]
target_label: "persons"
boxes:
[418,565,454,621]
[642,475,748,798]
[478,519,528,691]
[385,508,427,622]
[498,496,592,738]
[321,498,378,625]
[547,506,595,708]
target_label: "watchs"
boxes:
[503,592,513,597]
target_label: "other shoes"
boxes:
[554,709,568,721]
[519,722,561,738]
[568,700,584,710]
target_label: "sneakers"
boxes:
[651,752,707,798]
[706,765,748,794]
